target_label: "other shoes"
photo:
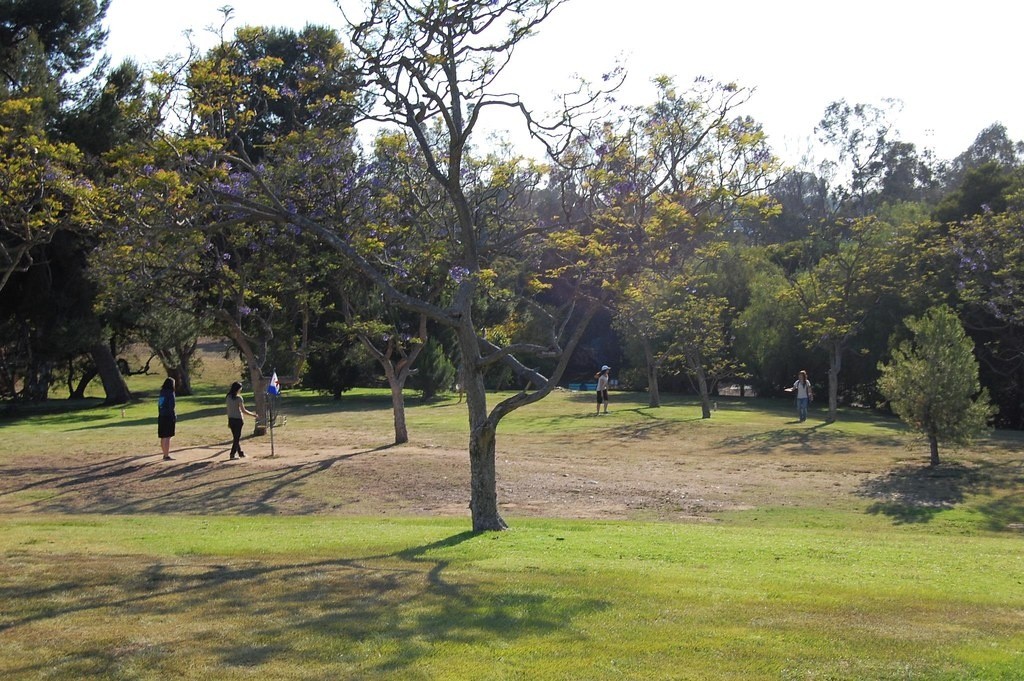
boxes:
[239,453,246,458]
[163,455,176,461]
[230,456,240,460]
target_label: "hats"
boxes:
[602,365,611,370]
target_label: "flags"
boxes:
[268,372,280,395]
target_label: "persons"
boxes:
[784,371,814,423]
[594,365,612,417]
[225,381,258,460]
[158,377,178,461]
